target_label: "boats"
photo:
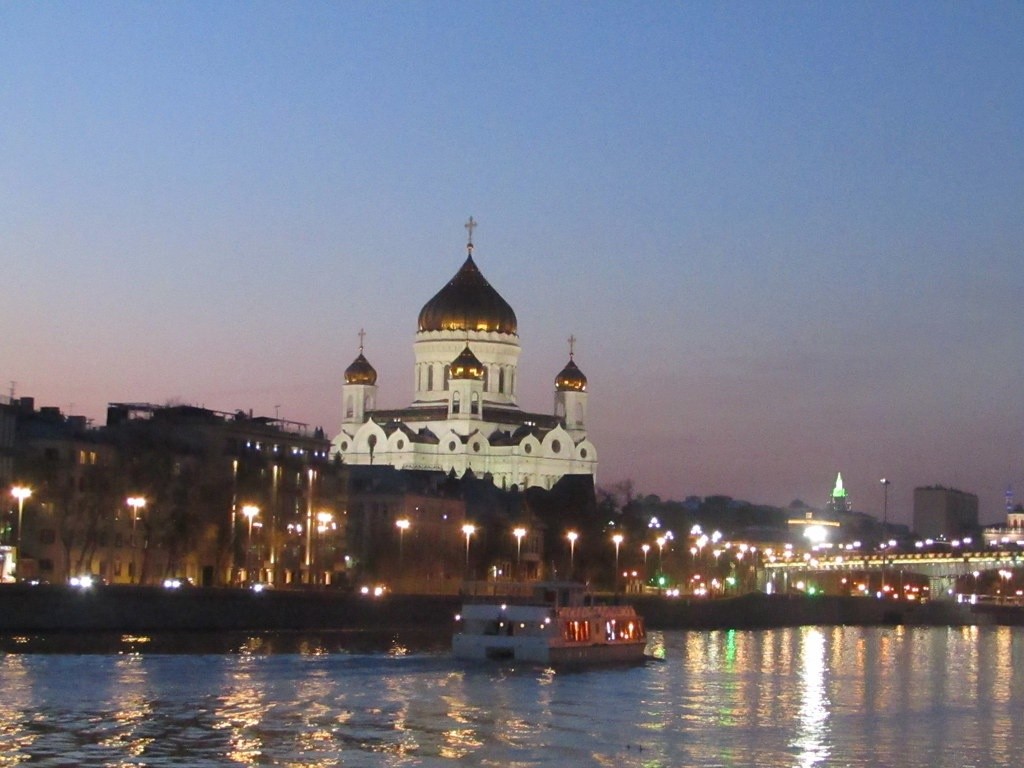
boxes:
[448,603,650,671]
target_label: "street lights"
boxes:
[244,505,258,589]
[567,531,577,567]
[127,497,146,584]
[462,523,476,596]
[880,477,893,594]
[513,527,525,580]
[10,485,33,584]
[319,511,332,584]
[613,534,624,597]
[396,518,410,590]
[656,535,667,578]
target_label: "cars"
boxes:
[360,581,396,597]
[163,576,196,588]
[248,581,275,591]
[69,571,111,587]
